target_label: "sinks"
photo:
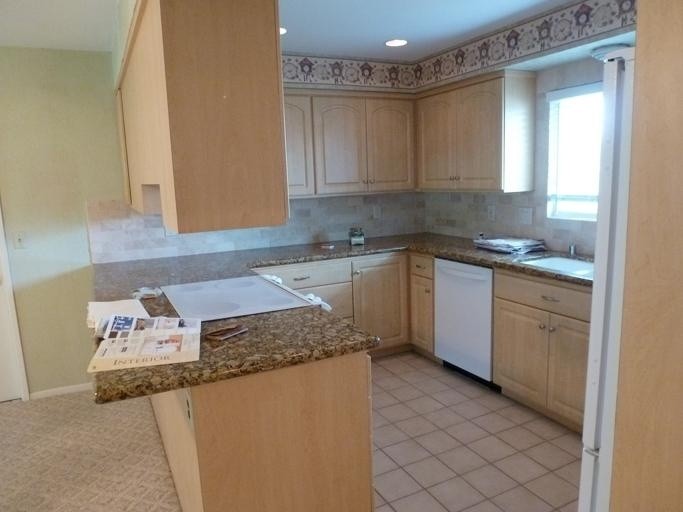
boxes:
[512,251,594,284]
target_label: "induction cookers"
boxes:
[160,275,314,322]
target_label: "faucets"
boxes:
[569,243,578,258]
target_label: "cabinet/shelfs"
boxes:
[493,267,592,438]
[281,92,417,203]
[349,251,410,355]
[407,252,434,355]
[250,258,354,326]
[417,69,535,194]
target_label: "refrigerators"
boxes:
[574,44,637,512]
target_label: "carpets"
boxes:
[1,386,182,512]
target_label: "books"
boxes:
[205,323,249,342]
[93,314,110,340]
